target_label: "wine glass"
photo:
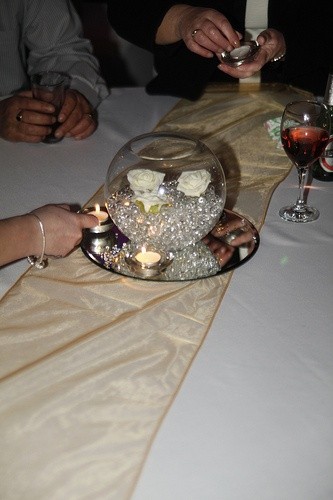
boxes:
[279,99,331,223]
[31,70,71,143]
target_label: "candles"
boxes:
[86,203,108,225]
[135,246,161,266]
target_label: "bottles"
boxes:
[313,78,333,182]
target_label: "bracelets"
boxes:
[269,50,286,62]
[25,213,46,269]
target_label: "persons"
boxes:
[106,0,333,102]
[0,204,99,267]
[0,0,109,144]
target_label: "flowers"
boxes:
[177,169,211,196]
[127,169,169,213]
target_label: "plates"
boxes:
[80,208,260,282]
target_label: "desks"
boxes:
[0,87,333,500]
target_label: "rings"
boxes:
[191,29,200,37]
[84,112,94,119]
[16,111,24,121]
